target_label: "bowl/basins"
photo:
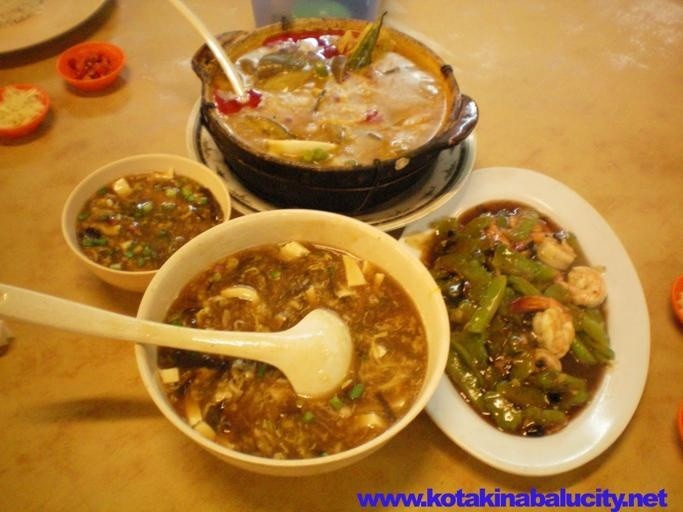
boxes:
[59,155,231,298]
[0,82,49,140]
[133,208,453,478]
[56,41,127,92]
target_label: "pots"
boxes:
[187,12,478,197]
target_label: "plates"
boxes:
[181,96,479,235]
[400,166,653,479]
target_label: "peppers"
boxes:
[432,210,615,433]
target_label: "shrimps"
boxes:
[552,266,609,309]
[532,305,576,359]
[536,236,577,270]
